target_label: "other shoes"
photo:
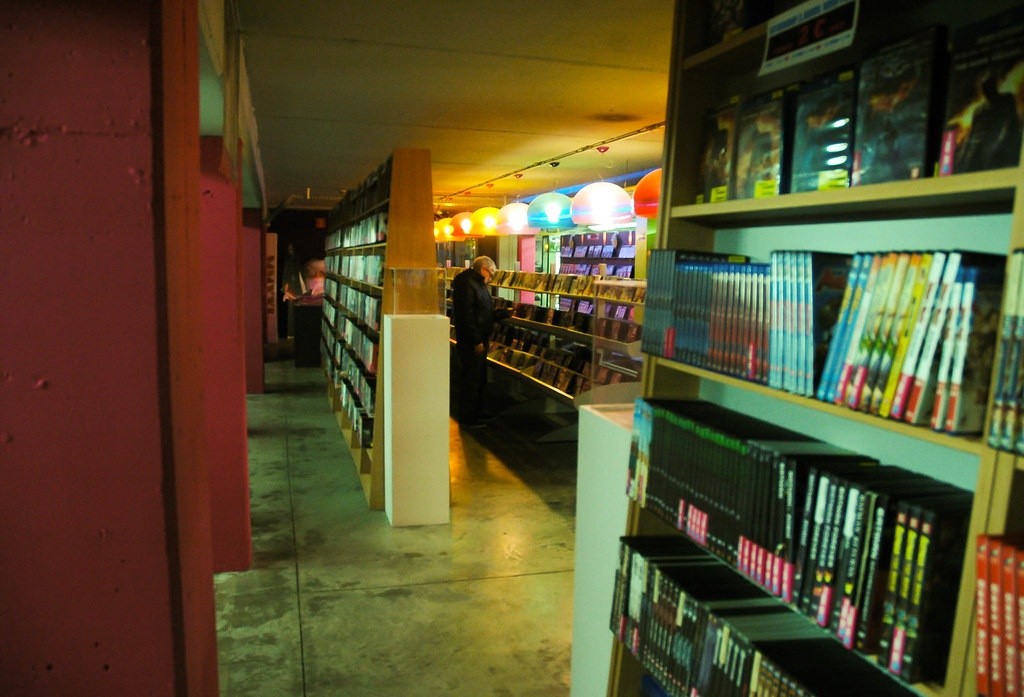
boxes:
[462,410,497,428]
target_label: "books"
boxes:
[695,0,1024,203]
[320,158,395,447]
[608,248,1022,697]
[560,231,635,278]
[445,267,647,400]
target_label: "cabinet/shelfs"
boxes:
[604,0,1024,697]
[319,148,454,513]
[446,275,646,445]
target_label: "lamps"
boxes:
[633,168,661,218]
[495,175,541,235]
[434,196,465,242]
[527,162,577,229]
[570,146,635,225]
[463,184,508,237]
[450,191,485,238]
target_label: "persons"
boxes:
[451,255,516,429]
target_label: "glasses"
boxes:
[483,266,495,279]
